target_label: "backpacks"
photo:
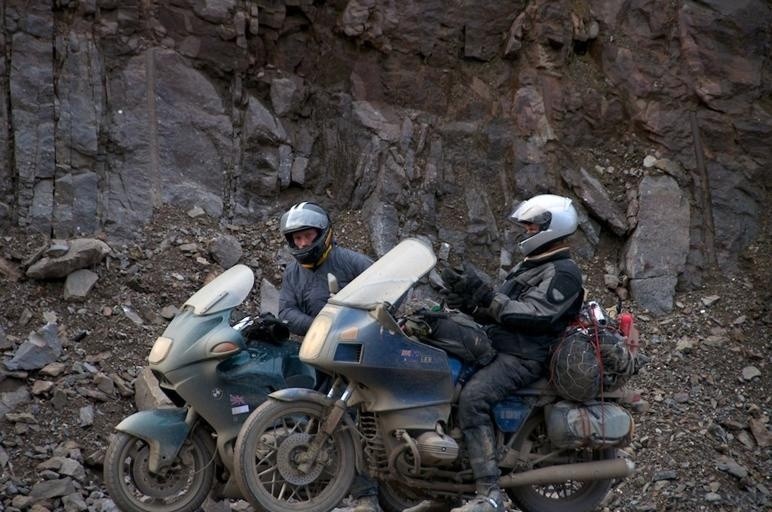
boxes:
[402,305,496,367]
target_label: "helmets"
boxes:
[280,201,333,269]
[507,193,579,257]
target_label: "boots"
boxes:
[450,425,505,512]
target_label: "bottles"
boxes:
[584,299,606,325]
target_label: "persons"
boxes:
[442,194,583,512]
[277,202,376,335]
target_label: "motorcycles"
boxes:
[101,260,469,512]
[232,233,646,511]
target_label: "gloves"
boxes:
[241,311,290,346]
[440,260,496,314]
